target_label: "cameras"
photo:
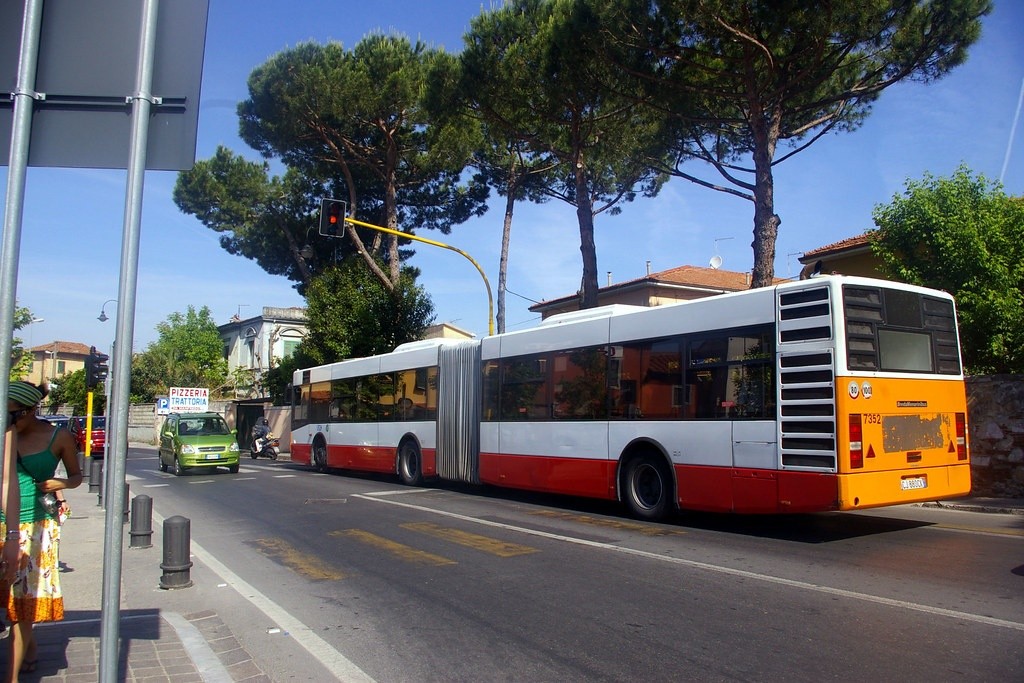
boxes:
[38,492,62,515]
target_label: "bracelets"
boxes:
[55,500,66,508]
[6,529,20,540]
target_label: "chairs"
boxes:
[202,423,215,430]
[180,422,188,434]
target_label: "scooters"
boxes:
[251,417,281,460]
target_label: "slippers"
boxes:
[19,659,38,673]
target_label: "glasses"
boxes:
[7,407,35,427]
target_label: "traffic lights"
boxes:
[320,197,346,240]
[89,353,108,387]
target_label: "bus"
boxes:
[286,277,972,523]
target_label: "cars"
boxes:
[68,415,108,458]
[37,414,68,427]
[159,411,241,474]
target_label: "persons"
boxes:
[199,418,221,432]
[254,419,271,453]
[179,423,187,435]
[0,380,83,683]
[0,414,20,583]
